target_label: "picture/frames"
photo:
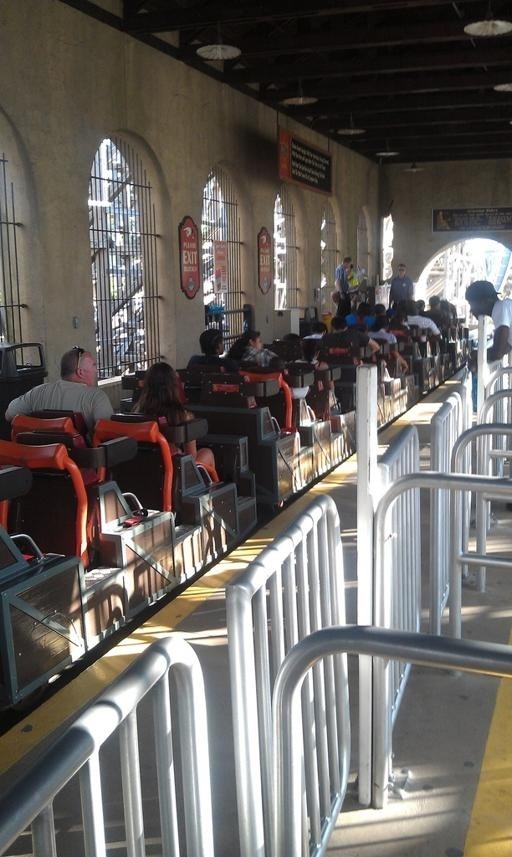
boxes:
[178,216,201,299]
[258,227,274,295]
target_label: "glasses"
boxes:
[71,345,83,368]
[398,268,405,271]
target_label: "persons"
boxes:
[225,331,279,368]
[131,362,216,471]
[465,281,512,372]
[283,256,461,415]
[187,329,239,373]
[5,346,115,438]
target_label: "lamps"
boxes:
[282,76,318,106]
[376,137,400,157]
[196,20,241,61]
[336,111,367,137]
[403,159,423,173]
[464,0,512,41]
[493,64,512,93]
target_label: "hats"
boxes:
[465,281,502,300]
